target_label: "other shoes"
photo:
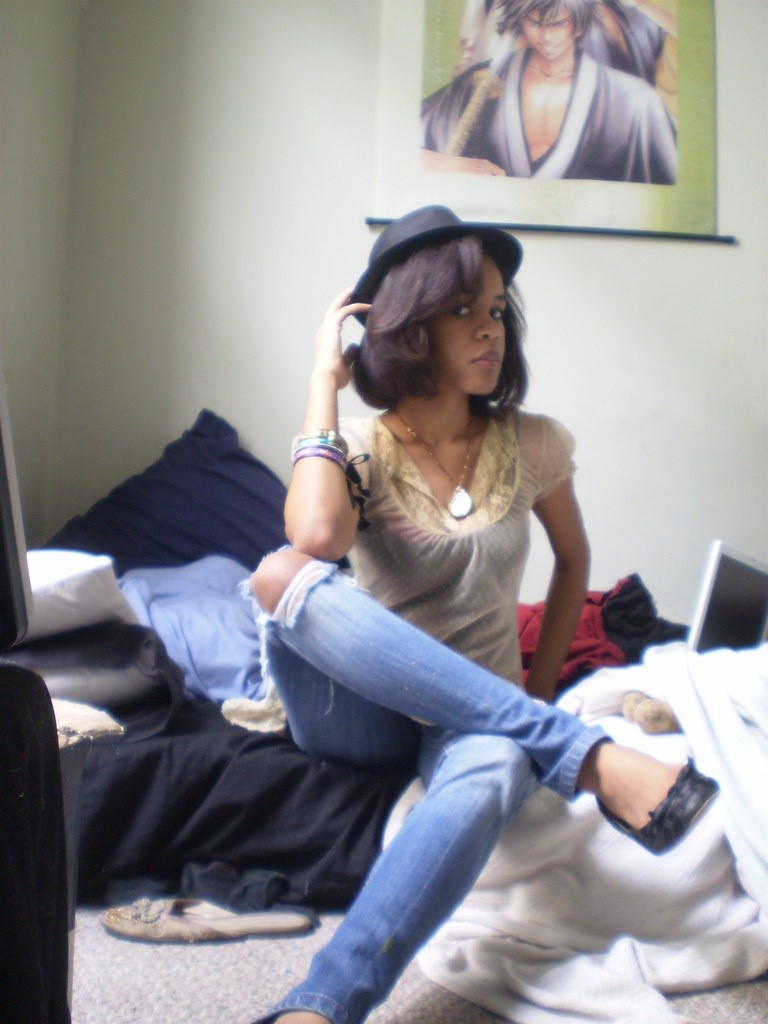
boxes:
[598,758,720,856]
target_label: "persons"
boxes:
[240,206,722,1024]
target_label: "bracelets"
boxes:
[293,439,346,469]
[294,428,349,449]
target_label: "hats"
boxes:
[346,204,524,326]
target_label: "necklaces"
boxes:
[398,415,474,519]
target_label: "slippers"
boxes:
[102,896,312,942]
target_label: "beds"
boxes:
[0,407,768,1024]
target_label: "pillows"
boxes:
[23,549,139,640]
